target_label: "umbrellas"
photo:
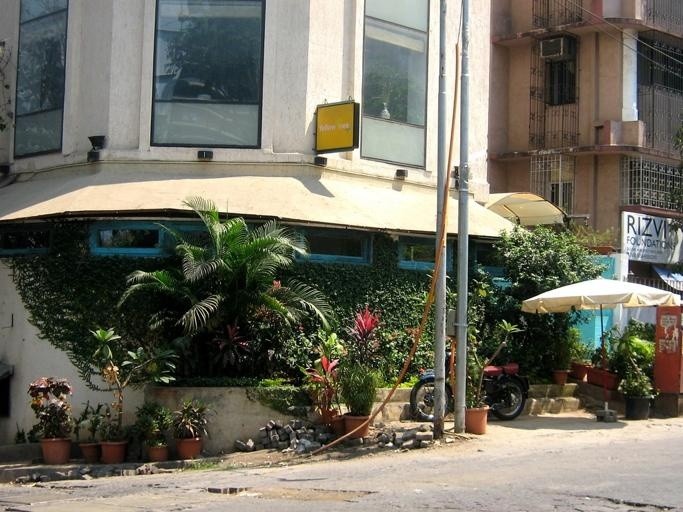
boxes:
[516,276,681,410]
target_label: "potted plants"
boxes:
[463,320,525,434]
[553,325,661,420]
[27,326,218,463]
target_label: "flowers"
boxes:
[296,305,379,439]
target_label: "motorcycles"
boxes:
[409,341,528,423]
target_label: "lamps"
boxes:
[86,135,105,161]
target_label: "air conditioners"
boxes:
[539,36,575,61]
